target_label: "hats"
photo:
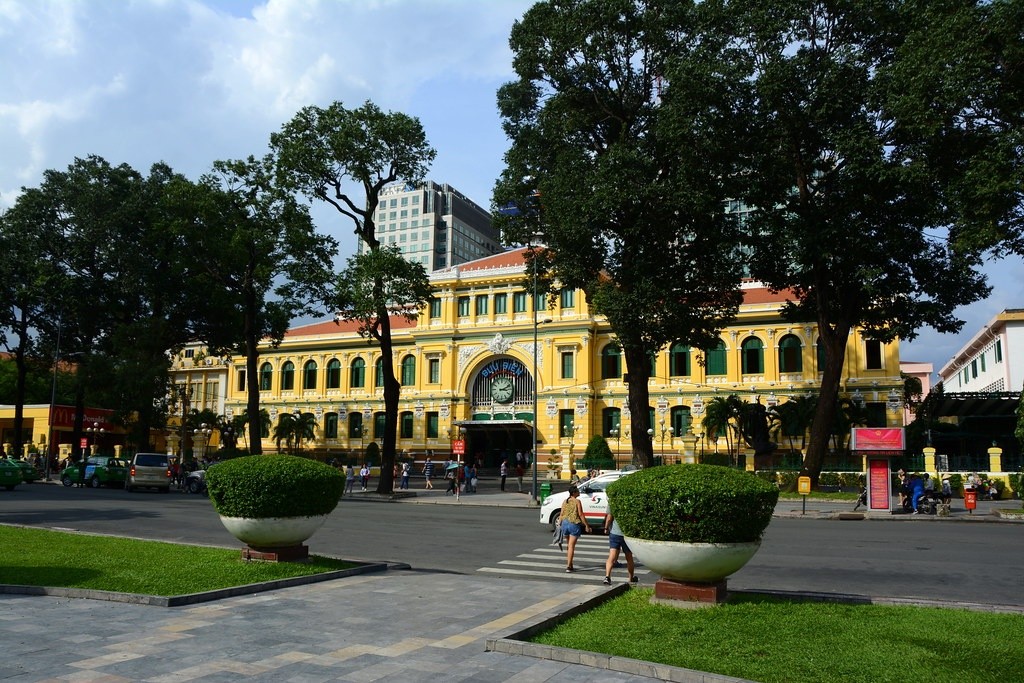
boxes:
[942,473,952,479]
[914,472,920,476]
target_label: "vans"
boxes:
[128,452,179,493]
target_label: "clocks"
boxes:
[491,377,514,404]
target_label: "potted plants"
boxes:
[605,463,780,582]
[204,455,348,548]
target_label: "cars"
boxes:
[0,458,24,491]
[11,459,41,484]
[59,454,130,489]
[186,469,206,494]
[538,472,634,533]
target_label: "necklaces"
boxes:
[514,464,525,493]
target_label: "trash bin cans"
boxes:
[964,488,977,513]
[540,483,553,503]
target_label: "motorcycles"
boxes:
[902,484,933,514]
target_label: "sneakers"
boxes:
[912,511,919,515]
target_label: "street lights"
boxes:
[531,317,554,502]
[87,422,106,444]
[647,418,675,467]
[194,422,213,459]
[355,422,369,468]
[609,423,630,471]
[446,420,467,502]
[700,430,706,464]
[563,420,584,444]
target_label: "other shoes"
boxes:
[566,566,575,573]
[604,577,611,584]
[631,576,639,582]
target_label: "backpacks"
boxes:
[406,465,412,474]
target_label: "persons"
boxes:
[570,469,579,486]
[500,459,510,492]
[896,469,952,516]
[971,479,995,500]
[359,463,370,492]
[400,460,410,491]
[77,457,100,488]
[393,463,398,489]
[589,469,596,480]
[344,463,356,494]
[24,451,73,471]
[422,458,435,490]
[602,505,638,584]
[170,456,221,493]
[516,451,523,461]
[559,486,592,573]
[443,458,478,496]
